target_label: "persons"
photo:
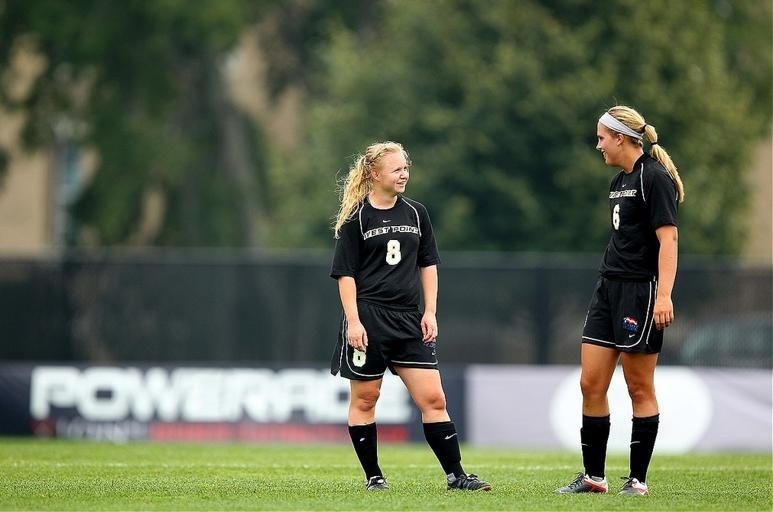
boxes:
[331,143,489,491]
[554,101,685,496]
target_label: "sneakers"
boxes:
[617,476,649,496]
[364,474,389,491]
[445,472,491,492]
[551,473,608,495]
